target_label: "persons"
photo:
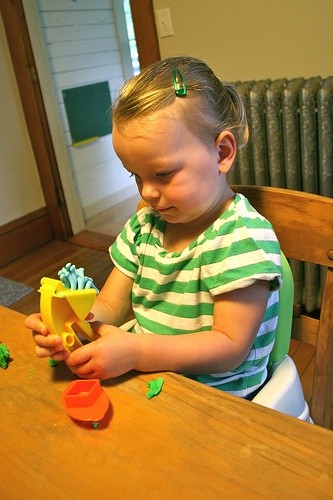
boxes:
[22,56,283,403]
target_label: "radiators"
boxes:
[227,75,333,312]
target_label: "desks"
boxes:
[0,306,333,500]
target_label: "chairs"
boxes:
[135,185,333,429]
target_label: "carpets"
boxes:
[0,276,34,306]
